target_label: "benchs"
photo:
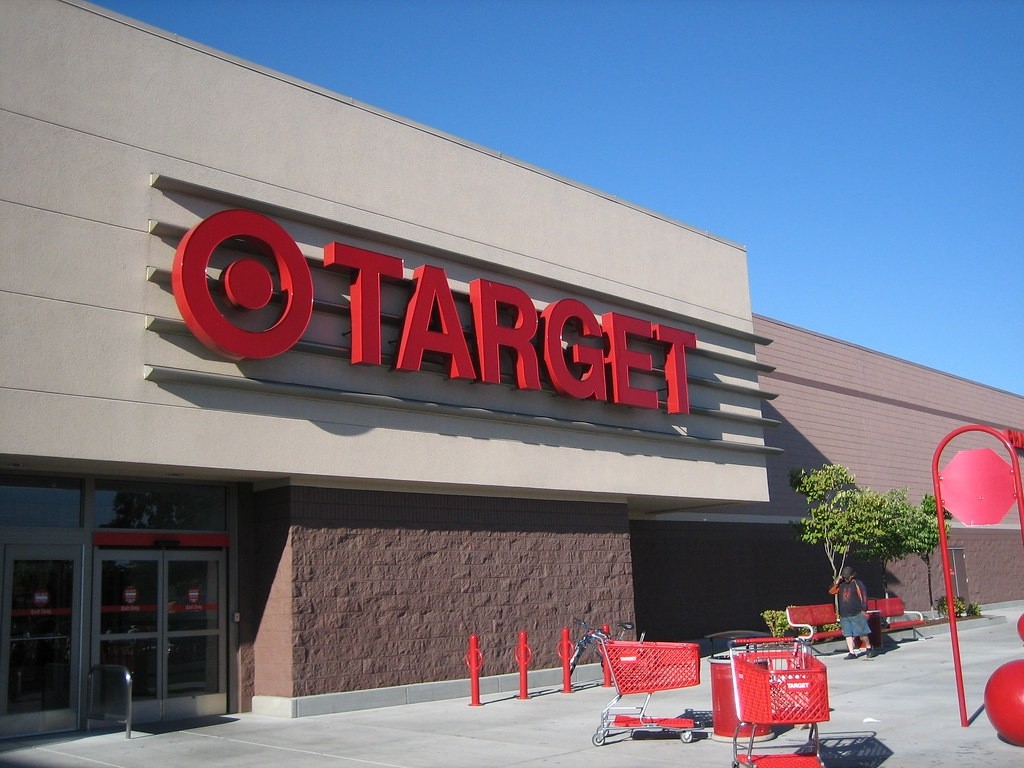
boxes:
[786,604,843,657]
[868,598,925,642]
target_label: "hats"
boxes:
[842,566,853,577]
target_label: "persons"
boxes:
[827,566,875,659]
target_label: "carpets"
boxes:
[123,715,240,736]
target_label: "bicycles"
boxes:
[567,617,646,677]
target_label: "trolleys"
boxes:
[586,627,702,745]
[726,635,833,768]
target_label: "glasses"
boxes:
[842,576,850,580]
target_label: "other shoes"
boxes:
[866,644,873,657]
[844,653,856,659]
